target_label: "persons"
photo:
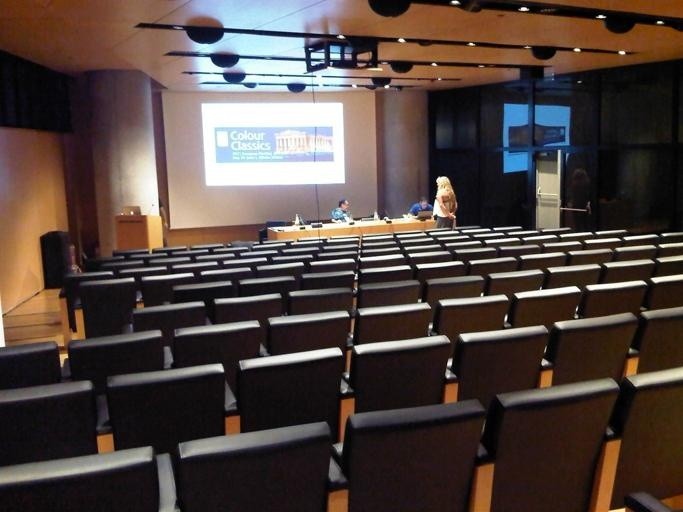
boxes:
[331,198,353,223]
[433,176,458,229]
[565,167,593,231]
[408,195,433,219]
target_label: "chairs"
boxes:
[0,223,682,512]
[258,220,286,243]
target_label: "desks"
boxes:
[265,217,439,243]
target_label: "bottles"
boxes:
[295,213,299,226]
[373,209,378,220]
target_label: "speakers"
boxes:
[40,231,72,289]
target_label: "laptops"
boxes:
[417,211,432,217]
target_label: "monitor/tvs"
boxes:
[122,206,141,216]
